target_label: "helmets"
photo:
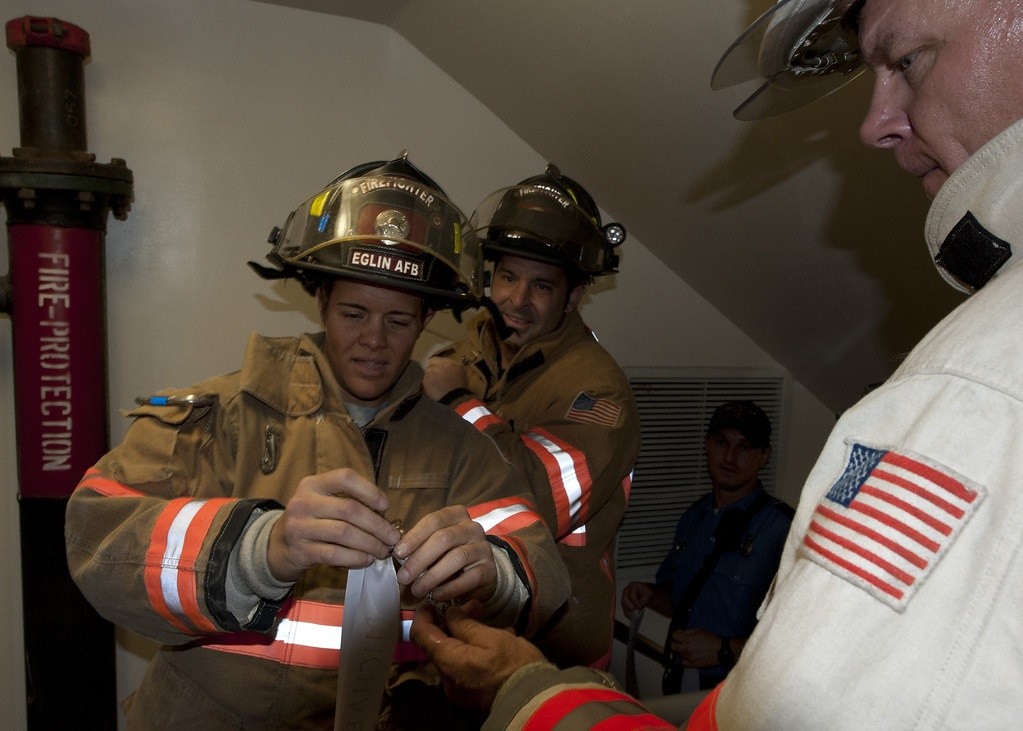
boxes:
[711,0,874,122]
[246,149,482,323]
[463,162,628,280]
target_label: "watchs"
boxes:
[718,637,735,665]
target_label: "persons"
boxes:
[61,145,574,731]
[402,162,635,688]
[620,395,797,697]
[411,1,1023,730]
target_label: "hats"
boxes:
[708,401,771,447]
